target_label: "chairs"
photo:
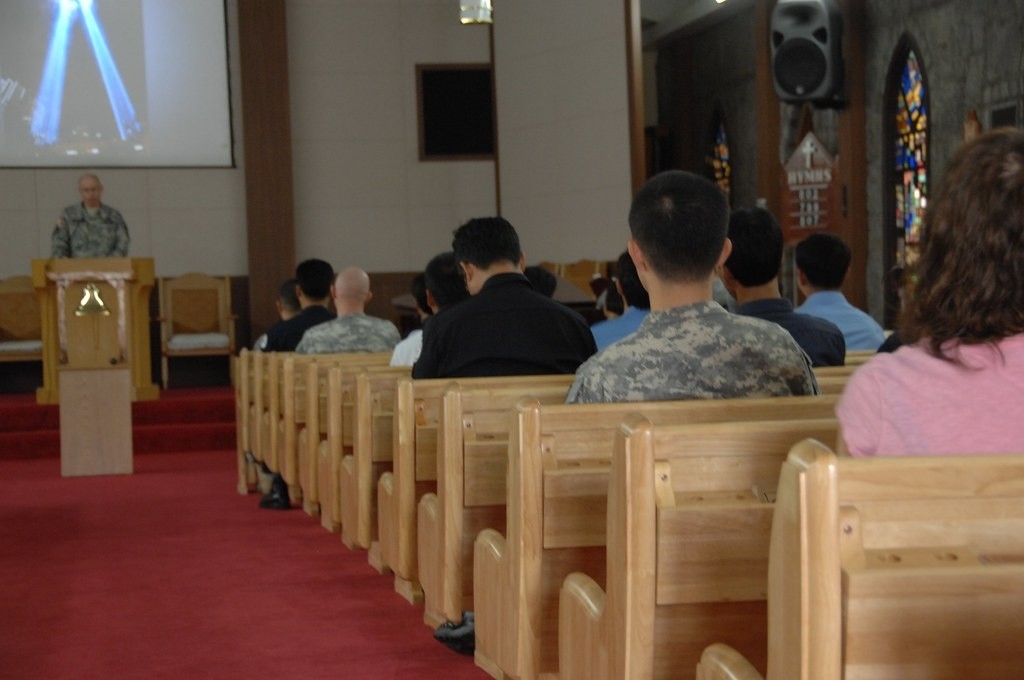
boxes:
[0,275,44,363]
[155,273,243,390]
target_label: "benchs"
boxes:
[229,328,1024,680]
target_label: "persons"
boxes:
[792,234,886,351]
[424,250,470,314]
[590,250,651,351]
[713,194,846,368]
[411,216,598,379]
[258,267,401,510]
[253,259,337,352]
[836,128,1024,457]
[52,174,130,258]
[524,265,557,297]
[433,171,821,657]
[390,273,433,367]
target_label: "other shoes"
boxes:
[434,610,476,656]
[260,479,289,510]
